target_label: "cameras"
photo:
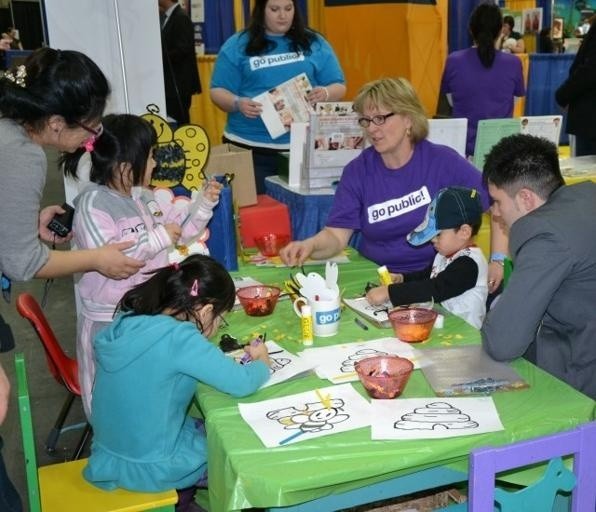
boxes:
[46,201,75,236]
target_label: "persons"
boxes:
[0,1,21,52]
[361,185,489,334]
[0,49,146,418]
[212,1,349,195]
[439,0,596,156]
[280,74,509,311]
[482,131,595,406]
[81,254,276,492]
[63,112,229,414]
[158,0,204,132]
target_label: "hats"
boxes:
[406,185,482,247]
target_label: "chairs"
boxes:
[13,352,179,512]
[431,422,596,512]
[502,257,516,292]
[16,292,93,460]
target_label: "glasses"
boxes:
[358,113,398,128]
[69,115,104,138]
[202,299,229,329]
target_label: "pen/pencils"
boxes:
[239,335,263,365]
[180,213,192,227]
[332,372,358,379]
[315,390,330,411]
[355,317,368,330]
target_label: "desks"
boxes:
[188,241,596,512]
[472,176,596,263]
[264,175,336,241]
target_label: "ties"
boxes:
[160,15,166,28]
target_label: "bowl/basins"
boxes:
[391,309,437,344]
[236,284,281,317]
[357,357,412,400]
[254,233,293,256]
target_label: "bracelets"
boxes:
[323,87,330,101]
[490,252,509,267]
[233,95,240,111]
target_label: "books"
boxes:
[252,71,319,140]
[287,101,562,190]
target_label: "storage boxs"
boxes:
[203,143,258,209]
[239,194,292,249]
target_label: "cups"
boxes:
[293,297,342,336]
